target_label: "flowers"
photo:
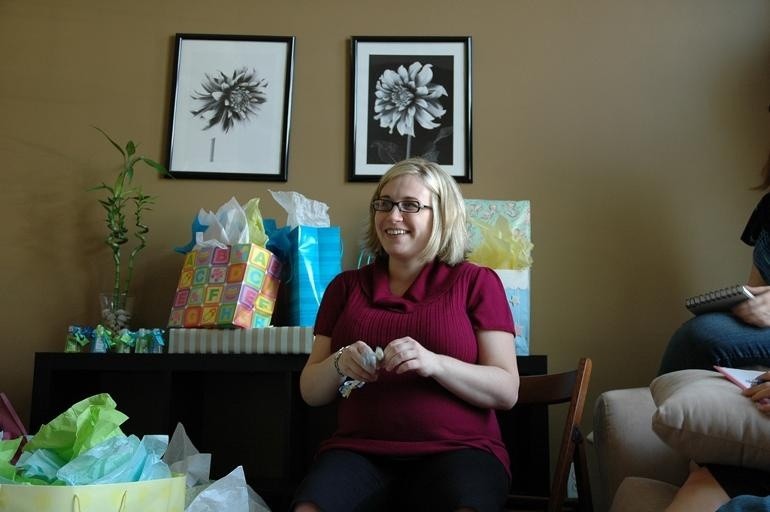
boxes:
[190,64,270,163]
[371,60,449,162]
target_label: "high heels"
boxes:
[166,225,344,329]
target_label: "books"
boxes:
[685,284,753,317]
[713,365,766,389]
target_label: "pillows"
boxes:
[645,367,769,468]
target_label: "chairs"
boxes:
[486,353,593,511]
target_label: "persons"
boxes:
[741,372,770,413]
[657,154,770,375]
[292,158,519,512]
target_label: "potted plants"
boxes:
[79,115,175,332]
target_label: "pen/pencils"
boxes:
[745,378,765,385]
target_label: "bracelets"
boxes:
[334,345,352,378]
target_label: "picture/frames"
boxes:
[347,34,473,185]
[164,34,296,182]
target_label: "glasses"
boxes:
[370,199,432,213]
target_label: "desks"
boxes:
[29,350,553,512]
